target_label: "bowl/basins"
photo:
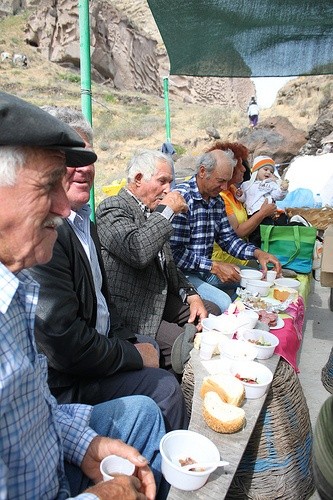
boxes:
[274,278,301,290]
[238,269,273,296]
[196,316,279,399]
[159,430,221,491]
[237,310,259,329]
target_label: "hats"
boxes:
[251,156,276,172]
[2,92,98,166]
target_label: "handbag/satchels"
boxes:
[258,225,317,273]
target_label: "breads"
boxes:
[273,288,298,301]
[201,373,245,406]
[204,391,246,433]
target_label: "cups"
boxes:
[267,271,277,286]
[100,455,135,481]
[160,193,184,216]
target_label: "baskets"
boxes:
[179,352,314,499]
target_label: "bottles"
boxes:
[314,194,322,209]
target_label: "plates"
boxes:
[251,309,284,329]
[272,287,299,294]
[269,268,297,278]
[260,298,280,306]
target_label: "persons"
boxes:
[205,141,289,269]
[95,150,221,385]
[247,96,259,126]
[167,148,282,312]
[0,92,157,500]
[33,105,189,434]
